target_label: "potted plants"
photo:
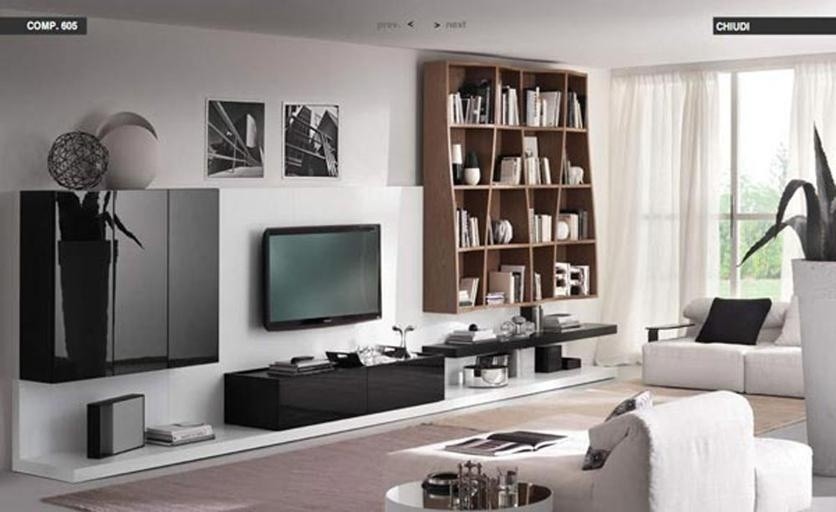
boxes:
[58,193,143,373]
[736,122,836,477]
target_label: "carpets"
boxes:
[39,422,484,511]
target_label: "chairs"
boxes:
[389,391,756,512]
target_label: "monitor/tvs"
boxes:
[263,223,382,331]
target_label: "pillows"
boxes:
[696,297,770,346]
[776,292,800,346]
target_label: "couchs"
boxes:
[643,296,802,397]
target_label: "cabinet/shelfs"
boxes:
[423,61,600,313]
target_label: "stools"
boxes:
[750,436,814,508]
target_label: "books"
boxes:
[449,85,583,129]
[267,359,334,377]
[494,137,583,185]
[445,330,497,344]
[145,423,215,446]
[445,431,568,457]
[459,262,589,307]
[456,208,588,247]
[543,314,584,333]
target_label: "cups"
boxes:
[530,305,543,332]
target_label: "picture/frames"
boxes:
[205,100,265,181]
[283,103,340,178]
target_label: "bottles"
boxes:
[496,470,518,509]
